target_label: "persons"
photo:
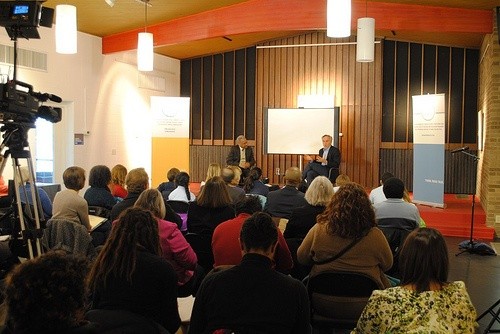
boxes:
[107,164,128,198]
[108,168,183,229]
[84,165,117,210]
[157,161,246,209]
[112,188,197,283]
[225,134,256,178]
[16,167,53,217]
[51,166,92,230]
[187,212,310,334]
[211,195,294,273]
[296,181,394,292]
[369,172,421,225]
[350,226,480,334]
[243,166,351,218]
[302,135,341,187]
[0,251,102,334]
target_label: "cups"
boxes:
[315,155,319,160]
[276,168,280,174]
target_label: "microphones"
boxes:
[48,94,62,103]
[451,146,469,153]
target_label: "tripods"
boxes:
[0,128,46,259]
[455,151,497,256]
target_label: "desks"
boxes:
[35,182,61,204]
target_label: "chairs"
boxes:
[305,268,383,334]
[42,218,98,260]
[166,199,189,233]
[86,310,171,334]
[185,233,213,264]
[196,265,236,295]
[284,237,312,283]
[374,218,419,279]
[86,206,111,247]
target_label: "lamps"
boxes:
[326,0,352,39]
[356,0,376,63]
[55,4,79,54]
[137,0,154,72]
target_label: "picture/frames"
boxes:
[477,109,483,151]
[74,134,84,145]
